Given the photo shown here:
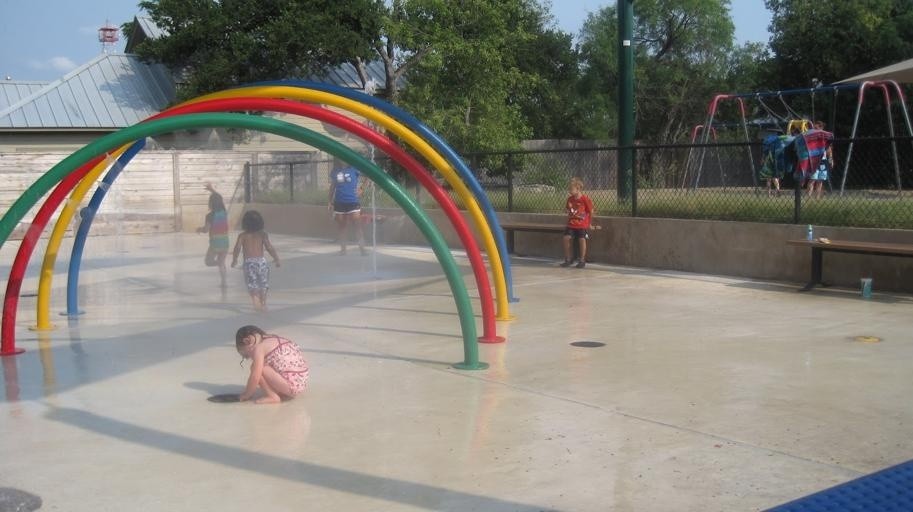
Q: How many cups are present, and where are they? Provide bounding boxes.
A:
[860,278,873,298]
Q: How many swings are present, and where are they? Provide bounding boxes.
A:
[721,125,741,185]
[756,90,837,170]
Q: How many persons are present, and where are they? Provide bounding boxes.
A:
[236,326,310,405]
[758,116,833,201]
[327,155,368,255]
[198,182,230,301]
[231,209,280,311]
[561,178,593,269]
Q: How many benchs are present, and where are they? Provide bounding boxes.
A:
[497,222,603,262]
[786,238,913,292]
[328,214,386,242]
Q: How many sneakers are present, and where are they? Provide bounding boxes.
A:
[560,262,571,267]
[576,262,585,269]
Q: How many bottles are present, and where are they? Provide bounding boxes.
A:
[807,224,813,240]
